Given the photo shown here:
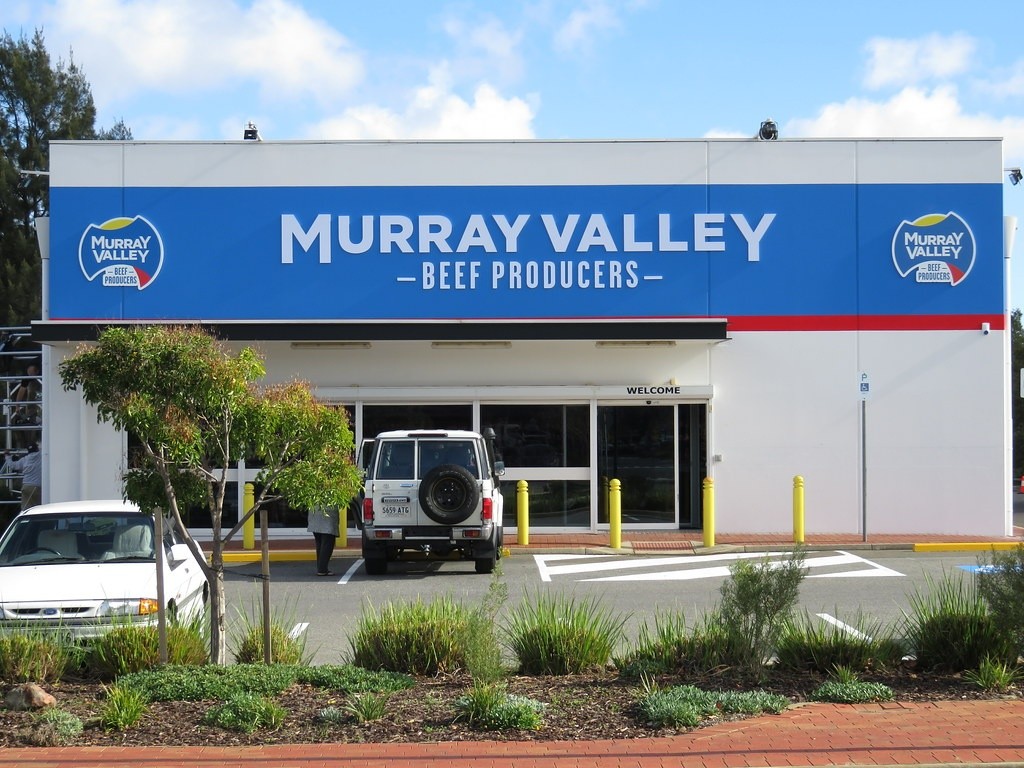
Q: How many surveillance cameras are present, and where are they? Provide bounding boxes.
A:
[762,125,775,139]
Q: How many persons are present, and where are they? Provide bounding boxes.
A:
[307,505,340,575]
[5,442,41,510]
[16,354,42,425]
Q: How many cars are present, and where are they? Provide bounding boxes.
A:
[0,500,210,652]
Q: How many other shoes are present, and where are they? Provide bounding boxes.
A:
[317,571,335,576]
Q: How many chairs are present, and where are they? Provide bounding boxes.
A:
[36,529,85,562]
[99,524,156,564]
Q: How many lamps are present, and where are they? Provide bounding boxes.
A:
[243,120,261,141]
[1004,167,1023,186]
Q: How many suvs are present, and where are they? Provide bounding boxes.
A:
[348,427,505,576]
